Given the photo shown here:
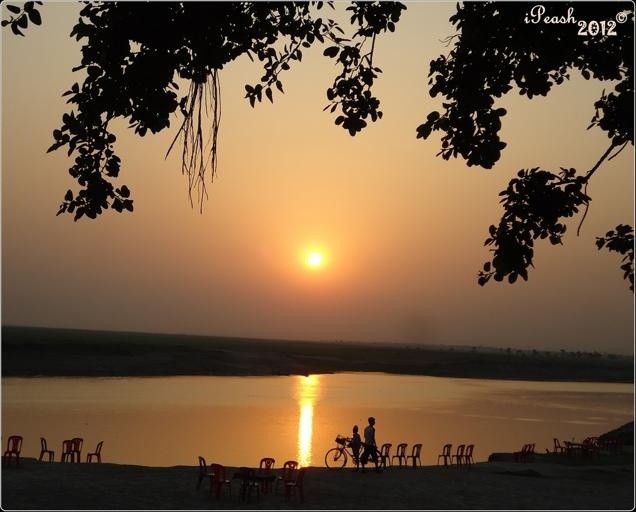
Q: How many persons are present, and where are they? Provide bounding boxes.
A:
[347,425,360,472]
[358,416,382,473]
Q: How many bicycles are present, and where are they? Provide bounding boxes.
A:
[325,435,383,469]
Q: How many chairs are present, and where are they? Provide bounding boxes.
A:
[238,467,260,504]
[208,463,232,500]
[407,444,423,468]
[380,444,392,468]
[286,468,305,504]
[260,458,275,495]
[462,445,474,465]
[438,444,452,466]
[1,434,104,464]
[392,443,408,468]
[275,461,298,496]
[196,456,214,490]
[510,437,622,464]
[452,445,465,464]
